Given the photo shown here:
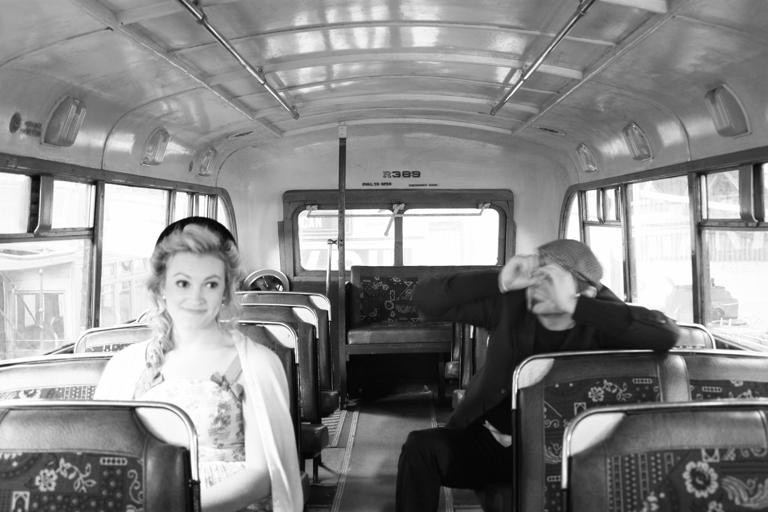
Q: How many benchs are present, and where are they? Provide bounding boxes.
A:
[444,294,765,512]
[0,265,330,512]
[340,266,511,396]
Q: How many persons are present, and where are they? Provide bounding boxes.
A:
[391,237,682,512]
[90,219,305,511]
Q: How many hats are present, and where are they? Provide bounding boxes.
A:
[153,215,236,251]
[537,239,603,291]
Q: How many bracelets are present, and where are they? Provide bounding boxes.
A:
[499,269,509,293]
